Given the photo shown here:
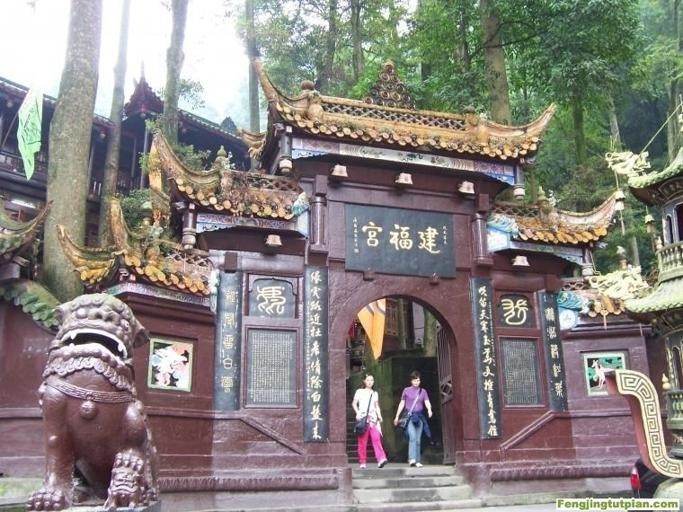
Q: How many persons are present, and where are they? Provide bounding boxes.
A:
[392,370,432,467]
[350,372,388,469]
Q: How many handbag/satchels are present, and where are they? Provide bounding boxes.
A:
[397,412,410,430]
[355,417,366,433]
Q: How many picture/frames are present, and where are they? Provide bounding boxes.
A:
[584,354,625,396]
[147,338,193,393]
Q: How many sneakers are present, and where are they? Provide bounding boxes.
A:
[360,464,366,469]
[377,459,387,468]
[409,459,423,468]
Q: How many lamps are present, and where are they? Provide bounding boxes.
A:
[263,232,282,248]
[329,162,476,196]
[510,254,530,267]
